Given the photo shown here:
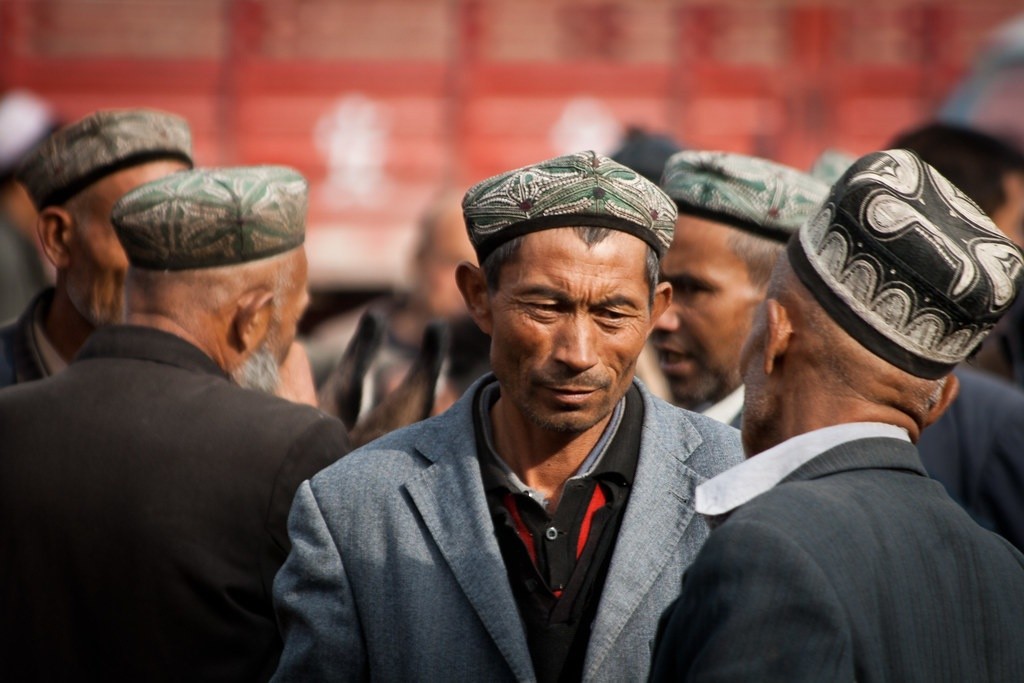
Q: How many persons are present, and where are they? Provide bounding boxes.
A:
[0,89,1023,543]
[646,147,1024,683]
[0,161,353,683]
[269,150,746,683]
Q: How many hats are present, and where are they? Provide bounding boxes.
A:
[14,111,193,212]
[655,149,831,243]
[0,90,63,180]
[787,147,1024,380]
[463,150,678,266]
[111,165,308,270]
